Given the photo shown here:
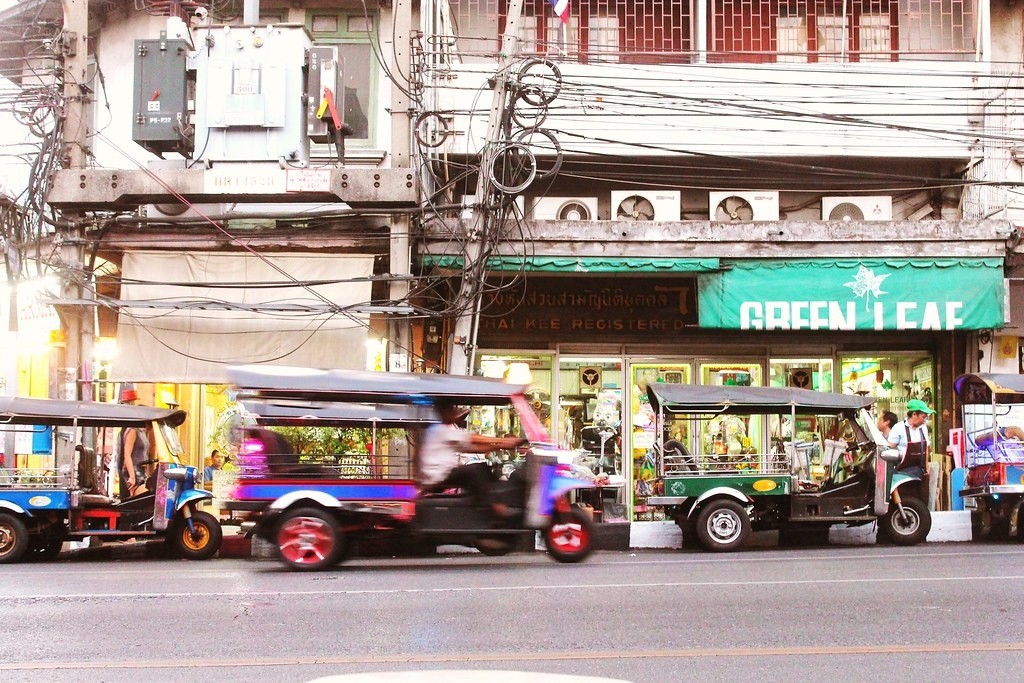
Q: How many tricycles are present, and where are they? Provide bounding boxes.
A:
[951,372,1023,545]
[0,395,224,563]
[226,360,605,571]
[645,382,933,553]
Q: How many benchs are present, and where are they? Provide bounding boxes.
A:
[664,439,698,476]
[251,426,338,479]
[976,426,1024,464]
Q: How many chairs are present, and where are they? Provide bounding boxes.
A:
[776,440,819,487]
[75,445,117,504]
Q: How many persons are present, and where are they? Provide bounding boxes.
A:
[875,399,937,546]
[418,408,525,518]
[877,410,898,442]
[118,406,152,544]
[204,450,240,482]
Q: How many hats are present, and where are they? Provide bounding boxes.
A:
[907,399,938,415]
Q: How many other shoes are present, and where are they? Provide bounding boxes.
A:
[499,507,523,516]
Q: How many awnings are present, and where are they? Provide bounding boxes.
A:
[113,251,370,383]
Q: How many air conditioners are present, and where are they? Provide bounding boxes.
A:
[531,196,598,223]
[460,194,524,221]
[820,195,892,222]
[609,190,681,224]
[708,190,779,224]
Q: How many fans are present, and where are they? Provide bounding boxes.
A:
[525,388,551,421]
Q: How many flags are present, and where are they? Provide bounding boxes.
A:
[549,0,570,24]
